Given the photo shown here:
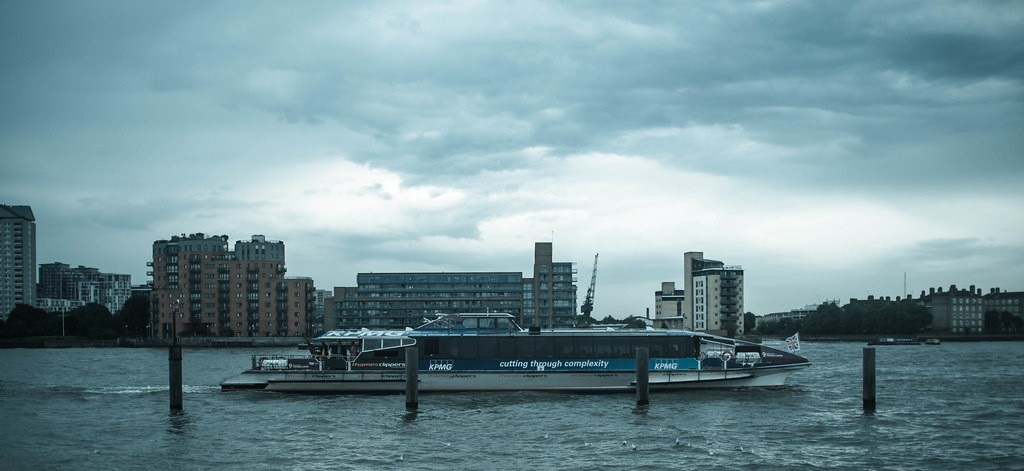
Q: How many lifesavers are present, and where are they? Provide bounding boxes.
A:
[258,356,268,367]
[722,351,733,362]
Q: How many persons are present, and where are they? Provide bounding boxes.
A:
[307,341,362,362]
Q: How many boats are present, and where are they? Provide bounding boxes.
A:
[926,338,940,345]
[220,307,811,393]
[867,336,922,345]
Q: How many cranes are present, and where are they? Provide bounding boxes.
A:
[581,253,600,320]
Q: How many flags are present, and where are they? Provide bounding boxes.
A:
[784,332,800,352]
